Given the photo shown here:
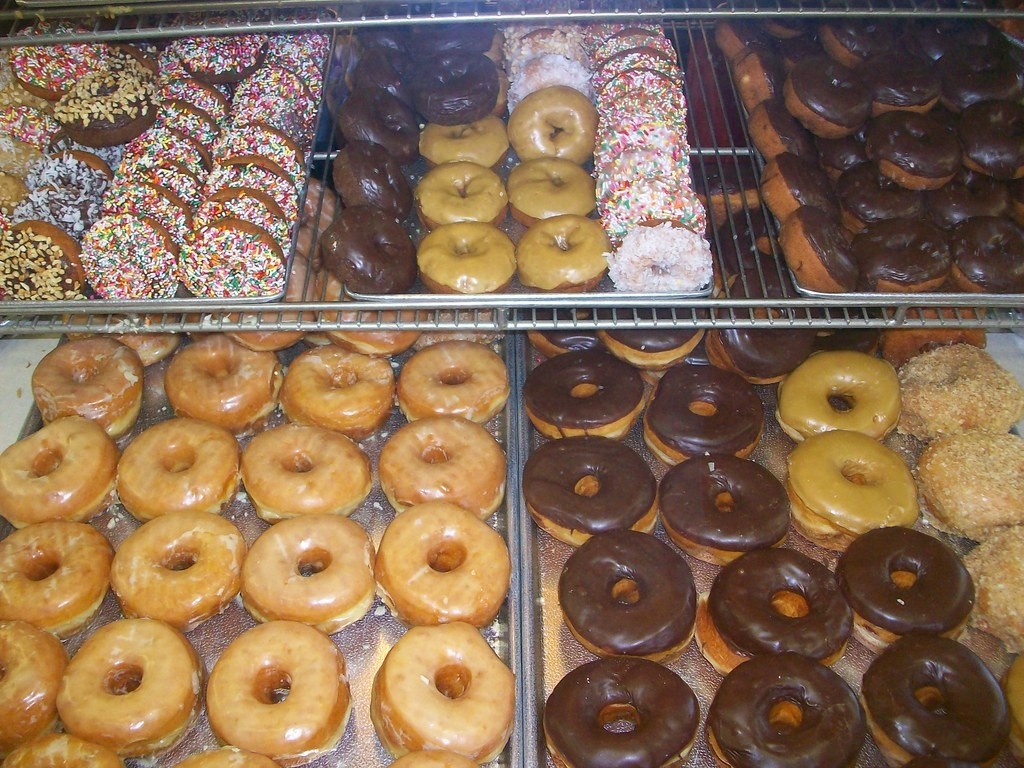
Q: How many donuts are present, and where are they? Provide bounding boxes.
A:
[0,0,1024,768]
